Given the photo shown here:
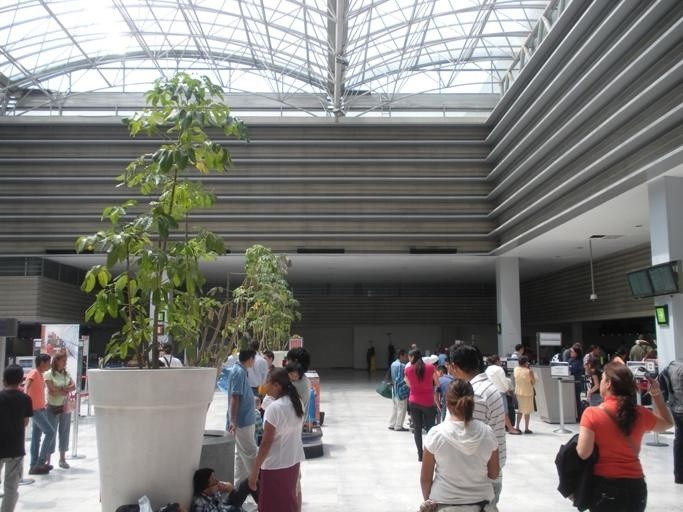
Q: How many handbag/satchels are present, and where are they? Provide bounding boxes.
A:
[510,394,517,409]
[375,381,394,398]
[62,389,76,413]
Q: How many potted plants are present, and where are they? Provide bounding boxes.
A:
[233,244,303,370]
[72,72,252,512]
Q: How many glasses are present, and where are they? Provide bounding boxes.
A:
[631,367,657,379]
[203,479,219,489]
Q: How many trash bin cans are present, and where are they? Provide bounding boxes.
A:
[197,429,236,486]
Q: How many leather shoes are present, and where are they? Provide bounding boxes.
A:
[395,426,409,431]
[389,426,394,430]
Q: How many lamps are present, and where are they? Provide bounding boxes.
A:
[589,239,598,303]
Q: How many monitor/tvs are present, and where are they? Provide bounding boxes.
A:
[656,306,668,323]
[648,260,679,296]
[626,269,654,298]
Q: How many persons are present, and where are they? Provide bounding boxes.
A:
[513,356,535,434]
[44,352,75,469]
[419,377,500,511]
[667,357,683,483]
[24,353,56,475]
[510,343,524,359]
[484,354,522,434]
[0,363,33,512]
[159,341,183,367]
[562,332,657,408]
[575,360,672,512]
[189,467,260,512]
[227,339,312,512]
[387,343,455,462]
[450,343,508,510]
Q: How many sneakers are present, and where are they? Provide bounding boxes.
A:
[417,452,423,461]
[18,477,35,485]
[59,460,68,468]
[29,466,49,473]
[675,477,683,483]
[406,419,427,433]
[0,483,5,497]
[33,463,52,471]
[509,428,532,435]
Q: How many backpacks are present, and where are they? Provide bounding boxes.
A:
[657,360,678,406]
[395,362,409,401]
